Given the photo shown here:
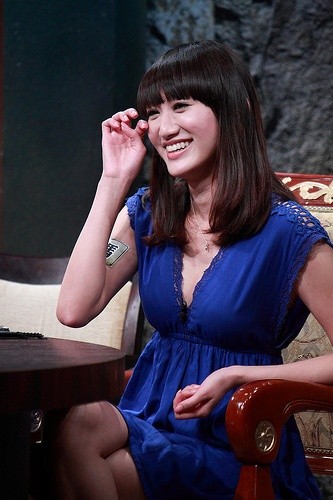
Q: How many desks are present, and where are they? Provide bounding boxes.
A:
[0,337,126,500]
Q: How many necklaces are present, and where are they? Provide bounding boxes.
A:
[189,196,212,251]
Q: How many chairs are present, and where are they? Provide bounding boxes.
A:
[121,173,333,500]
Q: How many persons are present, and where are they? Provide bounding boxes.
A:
[48,30,333,500]
